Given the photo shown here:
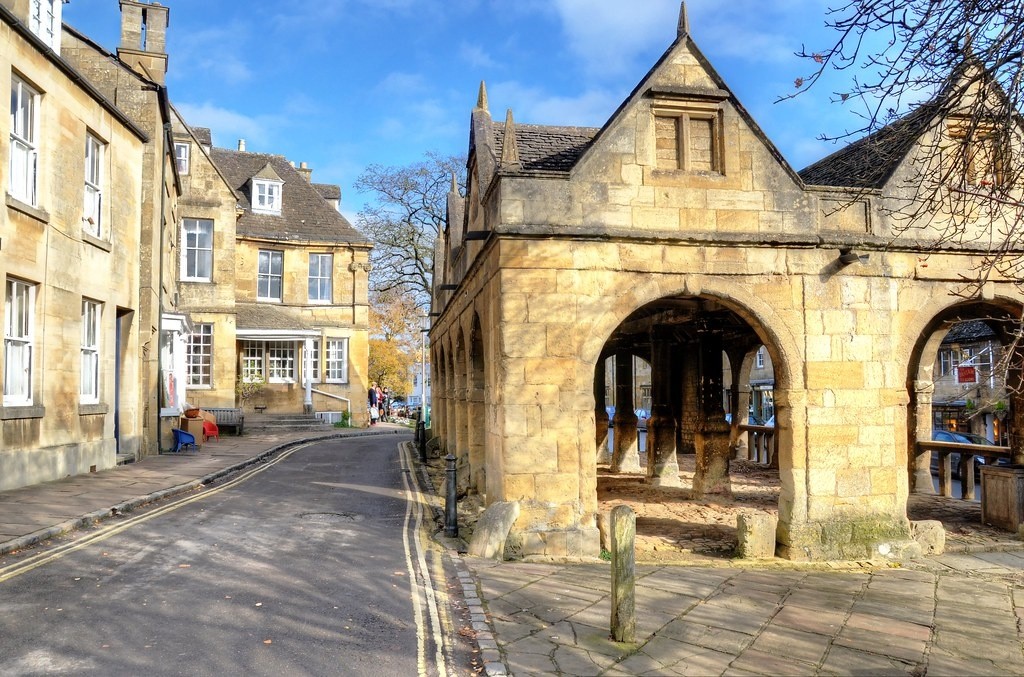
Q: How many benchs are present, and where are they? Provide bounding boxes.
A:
[197,407,244,435]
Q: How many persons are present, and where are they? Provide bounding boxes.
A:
[368,382,394,426]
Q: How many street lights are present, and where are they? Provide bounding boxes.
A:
[418,312,429,424]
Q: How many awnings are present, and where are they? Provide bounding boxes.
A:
[751,378,774,388]
[932,397,967,408]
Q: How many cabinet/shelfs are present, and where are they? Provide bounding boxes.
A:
[181,417,203,446]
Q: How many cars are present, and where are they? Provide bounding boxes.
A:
[634,409,652,432]
[604,406,617,427]
[391,401,431,420]
[931,429,1008,483]
[725,413,761,447]
[761,415,777,448]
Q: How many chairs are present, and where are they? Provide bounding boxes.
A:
[171,428,195,453]
[203,419,219,443]
